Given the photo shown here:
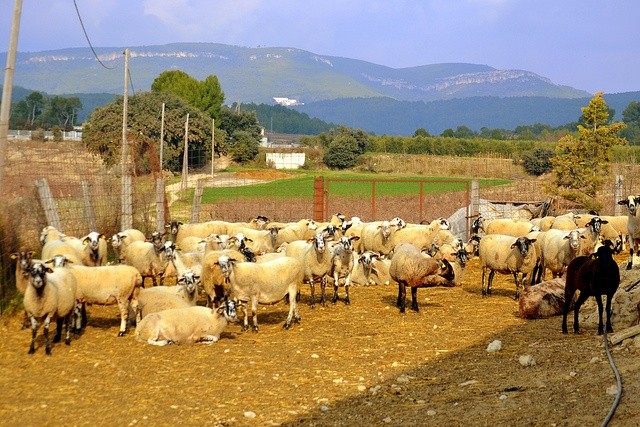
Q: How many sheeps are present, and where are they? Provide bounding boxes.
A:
[559,245,619,337]
[576,215,622,252]
[517,262,585,320]
[600,215,629,252]
[473,214,539,238]
[531,211,560,236]
[575,208,605,328]
[554,212,582,235]
[476,235,540,301]
[534,229,587,284]
[10,213,479,357]
[617,195,640,270]
